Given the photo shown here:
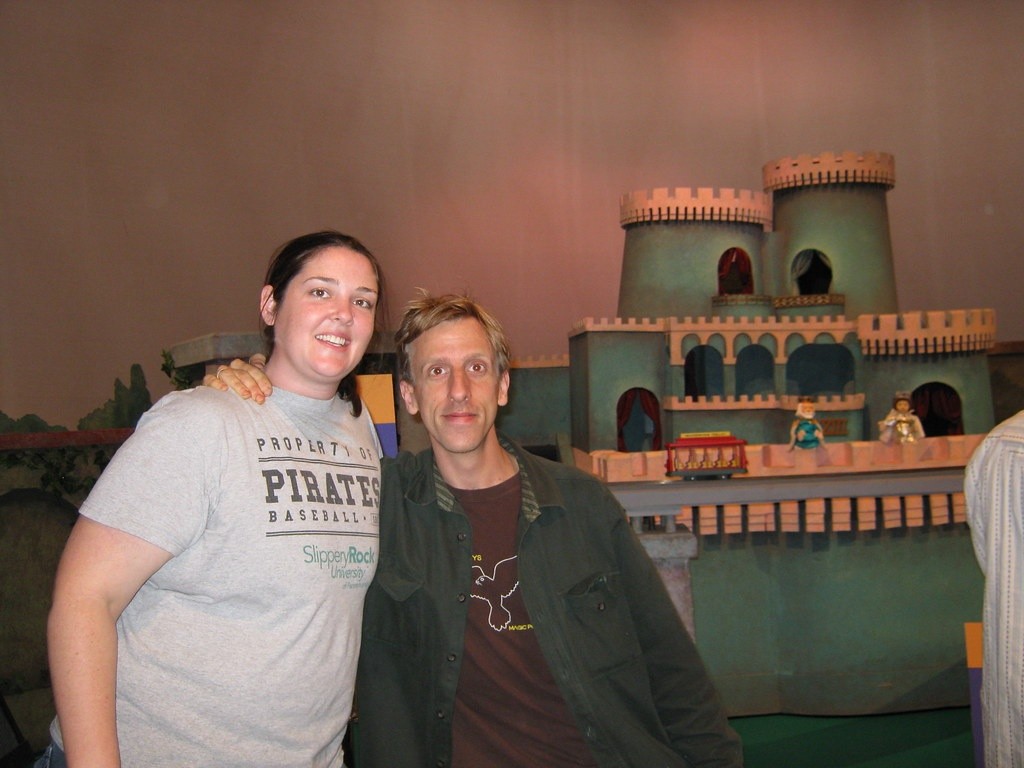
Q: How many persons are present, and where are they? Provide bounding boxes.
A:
[964,408,1024,768]
[786,395,827,452]
[876,391,926,446]
[202,288,740,768]
[46,229,384,768]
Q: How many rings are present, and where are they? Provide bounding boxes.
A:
[215,367,229,379]
